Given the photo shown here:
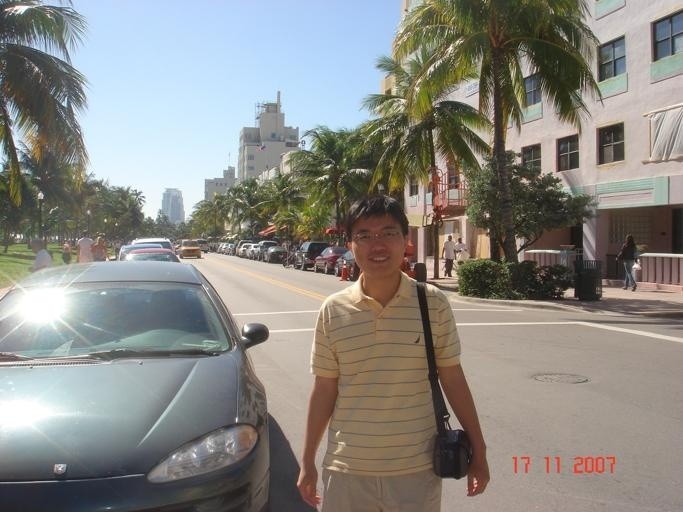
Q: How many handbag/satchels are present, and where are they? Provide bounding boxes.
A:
[433,428,472,479]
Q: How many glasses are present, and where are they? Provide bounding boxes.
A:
[353,229,404,243]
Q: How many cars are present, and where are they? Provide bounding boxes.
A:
[0,259,270,512]
[115,234,411,281]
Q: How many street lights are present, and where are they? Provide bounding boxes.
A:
[36,190,122,265]
[430,196,443,280]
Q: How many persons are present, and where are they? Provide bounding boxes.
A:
[28,233,137,273]
[616,234,639,292]
[441,235,456,277]
[295,196,491,512]
[454,237,468,266]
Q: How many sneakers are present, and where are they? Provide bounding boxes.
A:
[621,283,637,292]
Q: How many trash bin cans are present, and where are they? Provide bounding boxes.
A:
[572,260,603,302]
